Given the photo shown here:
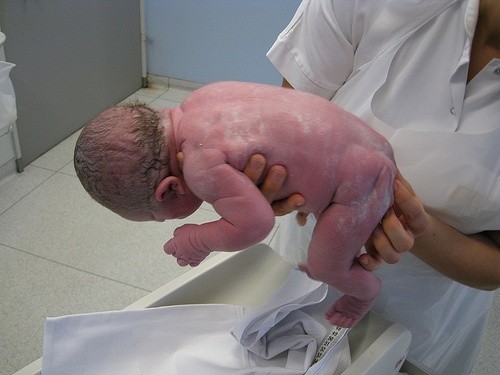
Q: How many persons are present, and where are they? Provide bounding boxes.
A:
[230,0,500,375]
[72,79,399,331]
